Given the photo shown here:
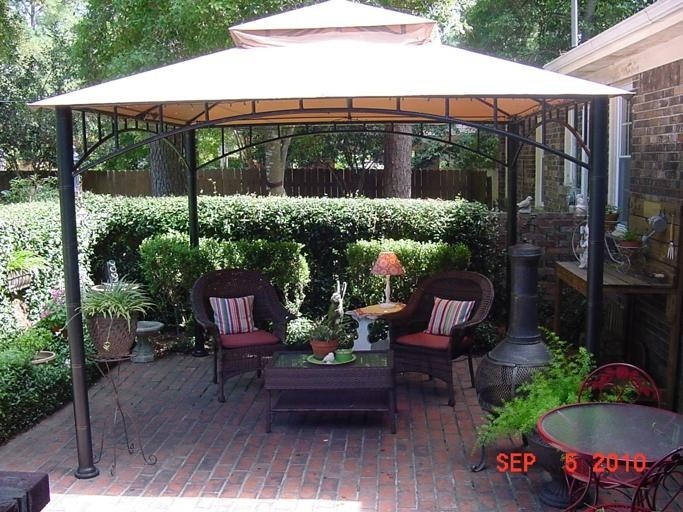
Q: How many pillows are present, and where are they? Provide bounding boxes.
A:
[208,295,258,334]
[424,295,475,337]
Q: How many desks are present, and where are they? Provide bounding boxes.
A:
[537,401,683,512]
[554,261,683,411]
[345,303,407,351]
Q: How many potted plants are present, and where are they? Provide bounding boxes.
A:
[470,326,650,509]
[604,204,641,256]
[282,317,354,362]
[4,250,44,293]
[58,272,157,360]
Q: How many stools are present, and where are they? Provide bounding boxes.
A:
[130,321,165,363]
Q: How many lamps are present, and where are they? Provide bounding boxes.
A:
[372,252,404,308]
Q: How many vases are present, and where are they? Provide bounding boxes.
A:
[31,350,55,364]
[52,326,67,339]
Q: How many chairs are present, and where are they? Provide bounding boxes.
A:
[192,268,292,402]
[564,363,661,512]
[587,446,683,512]
[388,271,495,406]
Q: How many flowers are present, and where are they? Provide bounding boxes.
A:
[40,287,67,328]
